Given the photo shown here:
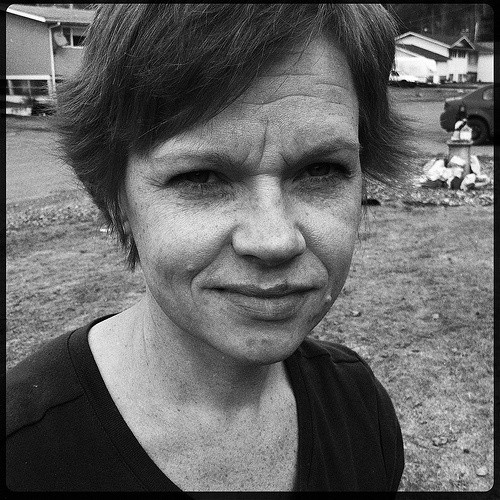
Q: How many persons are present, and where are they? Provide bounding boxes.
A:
[6,5,420,492]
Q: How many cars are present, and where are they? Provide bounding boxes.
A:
[439,85,494,145]
